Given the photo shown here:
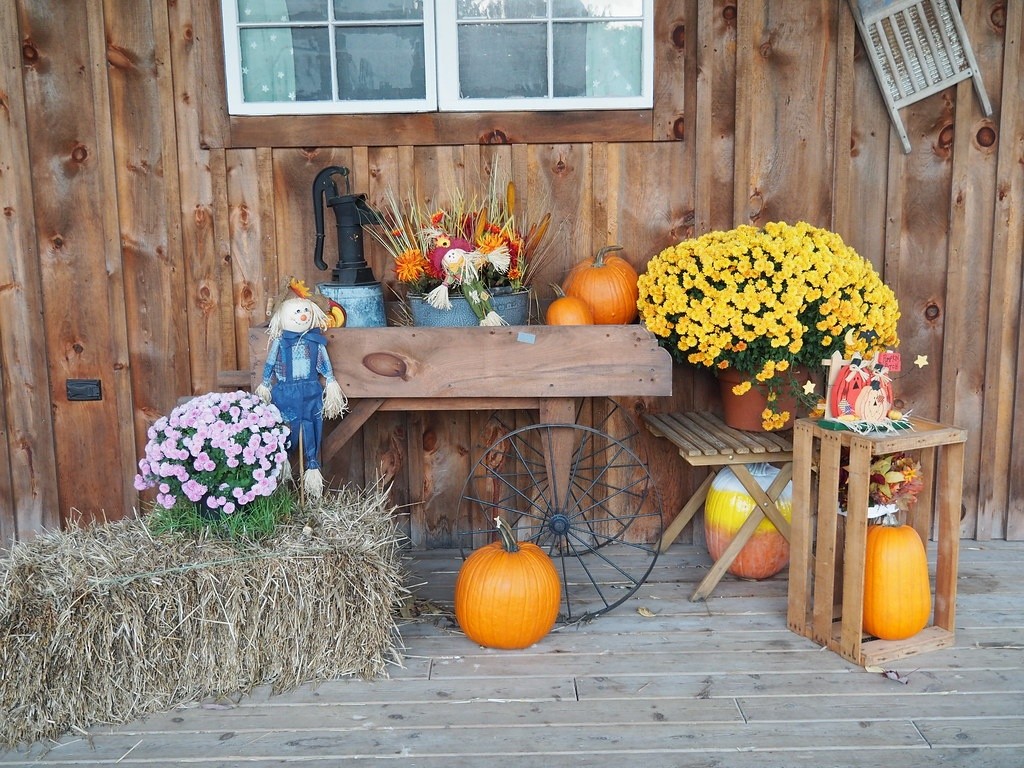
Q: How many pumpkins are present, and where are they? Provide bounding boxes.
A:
[545,245,642,326]
[863,518,933,639]
[705,462,792,578]
[454,516,563,650]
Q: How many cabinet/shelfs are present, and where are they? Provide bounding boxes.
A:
[642,406,793,603]
[787,411,970,669]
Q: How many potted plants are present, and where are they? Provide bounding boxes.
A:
[134,390,292,519]
[354,151,572,327]
[837,446,924,527]
[635,217,904,432]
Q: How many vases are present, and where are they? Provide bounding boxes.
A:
[319,280,387,326]
